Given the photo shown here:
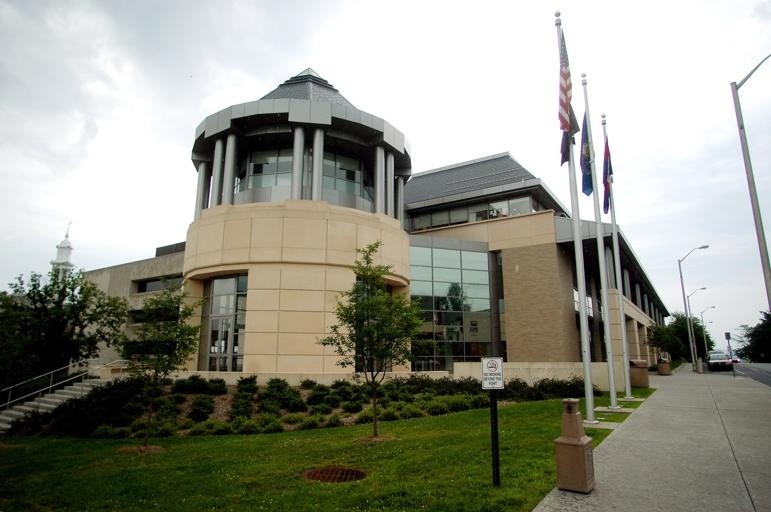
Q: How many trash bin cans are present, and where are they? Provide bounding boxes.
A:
[657,358,670,375]
[696,357,703,373]
[629,359,649,388]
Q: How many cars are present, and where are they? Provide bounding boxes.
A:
[707,349,741,373]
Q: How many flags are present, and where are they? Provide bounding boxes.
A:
[602,137,614,213]
[556,27,579,165]
[580,113,593,196]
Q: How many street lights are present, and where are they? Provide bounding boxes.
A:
[677,244,717,371]
